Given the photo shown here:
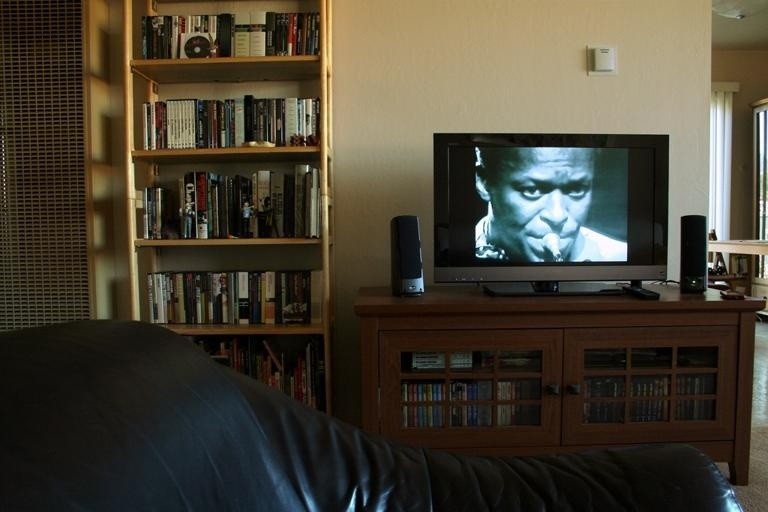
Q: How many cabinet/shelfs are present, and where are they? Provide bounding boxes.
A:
[120,0,333,419]
[354,285,767,489]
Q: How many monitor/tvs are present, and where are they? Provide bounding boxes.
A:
[434,132,669,296]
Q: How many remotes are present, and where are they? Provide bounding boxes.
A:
[624,286,659,300]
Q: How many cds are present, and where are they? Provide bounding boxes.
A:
[184,37,211,58]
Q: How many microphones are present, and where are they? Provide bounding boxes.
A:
[542,233,563,263]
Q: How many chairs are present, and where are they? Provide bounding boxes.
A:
[708,229,748,291]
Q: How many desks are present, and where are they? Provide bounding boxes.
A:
[708,239,768,256]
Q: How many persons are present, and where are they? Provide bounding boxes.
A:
[474,147,628,263]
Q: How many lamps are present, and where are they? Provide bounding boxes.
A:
[712,0,768,19]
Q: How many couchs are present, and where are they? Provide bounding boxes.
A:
[1,318,747,512]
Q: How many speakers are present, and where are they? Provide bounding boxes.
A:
[680,215,707,294]
[391,215,424,297]
